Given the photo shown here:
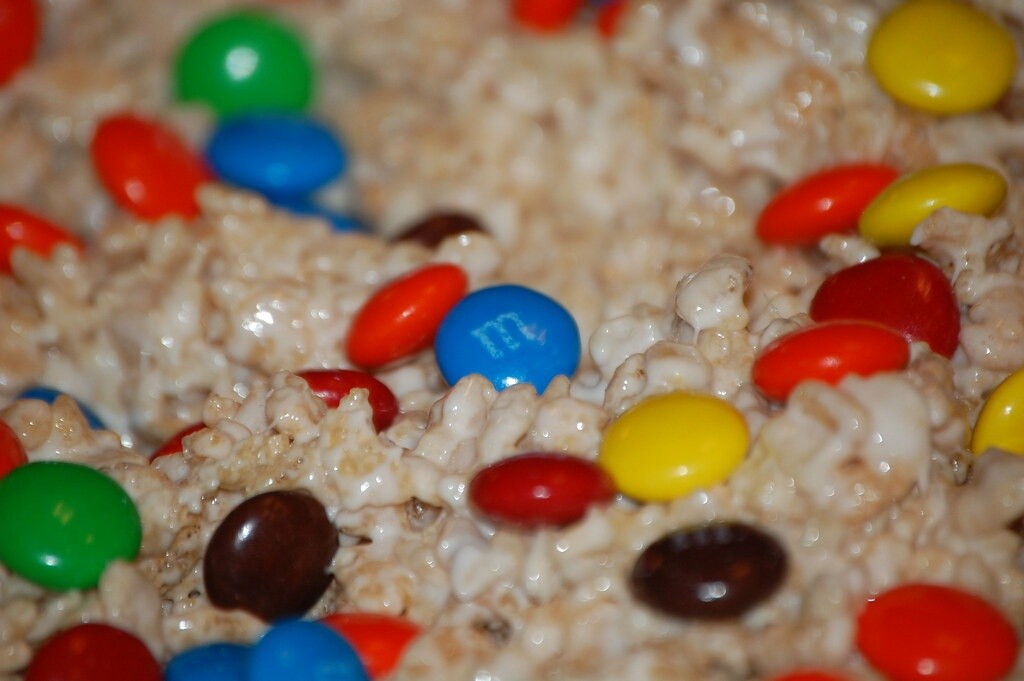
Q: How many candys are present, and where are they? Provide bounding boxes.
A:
[1,0,1024,681]
